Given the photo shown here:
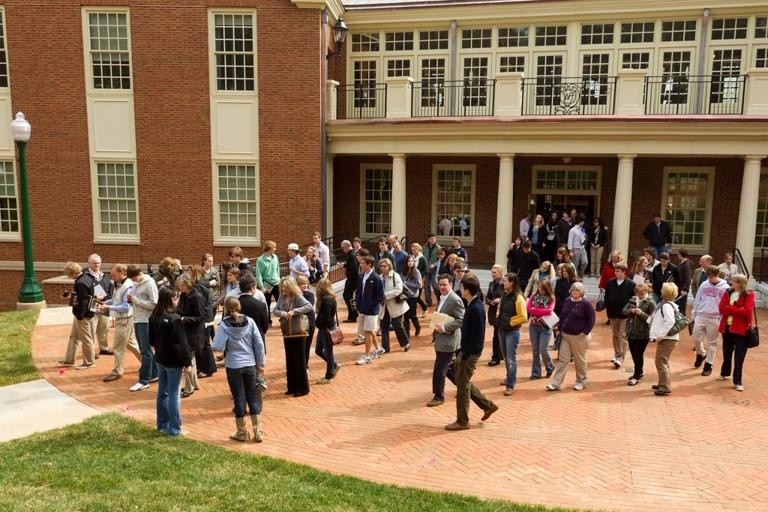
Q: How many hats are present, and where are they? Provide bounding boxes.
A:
[288,243,300,251]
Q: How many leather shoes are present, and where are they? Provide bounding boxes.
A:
[285,389,309,397]
[103,373,122,383]
[628,374,643,386]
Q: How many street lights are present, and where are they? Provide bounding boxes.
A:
[7,108,48,312]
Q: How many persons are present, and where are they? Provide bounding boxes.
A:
[643,213,672,259]
[59,254,141,381]
[520,208,607,281]
[126,254,220,436]
[691,253,755,390]
[485,237,596,395]
[342,235,429,365]
[423,233,499,430]
[256,233,341,396]
[213,247,268,441]
[440,214,470,236]
[599,248,693,395]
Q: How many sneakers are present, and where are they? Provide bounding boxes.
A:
[445,421,471,430]
[716,372,729,380]
[57,347,114,370]
[156,426,189,437]
[694,353,706,367]
[480,404,499,421]
[609,355,624,367]
[573,380,587,390]
[488,355,555,396]
[590,272,600,277]
[426,396,444,407]
[129,376,159,391]
[652,385,671,396]
[181,353,225,398]
[342,309,428,366]
[736,384,743,391]
[316,364,341,384]
[547,382,560,390]
[702,362,712,376]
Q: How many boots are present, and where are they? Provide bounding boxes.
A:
[250,413,266,443]
[230,416,249,442]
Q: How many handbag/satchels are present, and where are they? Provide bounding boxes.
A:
[595,300,606,312]
[328,327,343,345]
[537,310,560,331]
[310,271,323,283]
[394,281,419,303]
[280,313,309,337]
[745,326,759,348]
[665,313,690,336]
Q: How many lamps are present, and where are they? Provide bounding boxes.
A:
[326,15,349,60]
